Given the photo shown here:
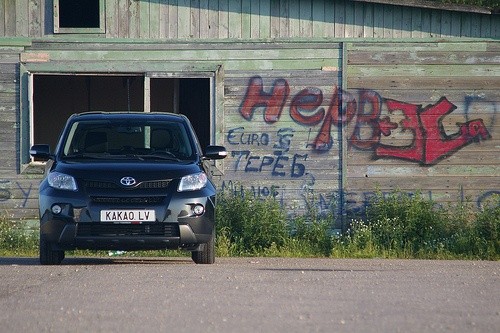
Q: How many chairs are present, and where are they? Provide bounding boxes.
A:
[82,132,108,154]
[150,128,172,152]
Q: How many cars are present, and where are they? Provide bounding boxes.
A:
[28,110,228,264]
